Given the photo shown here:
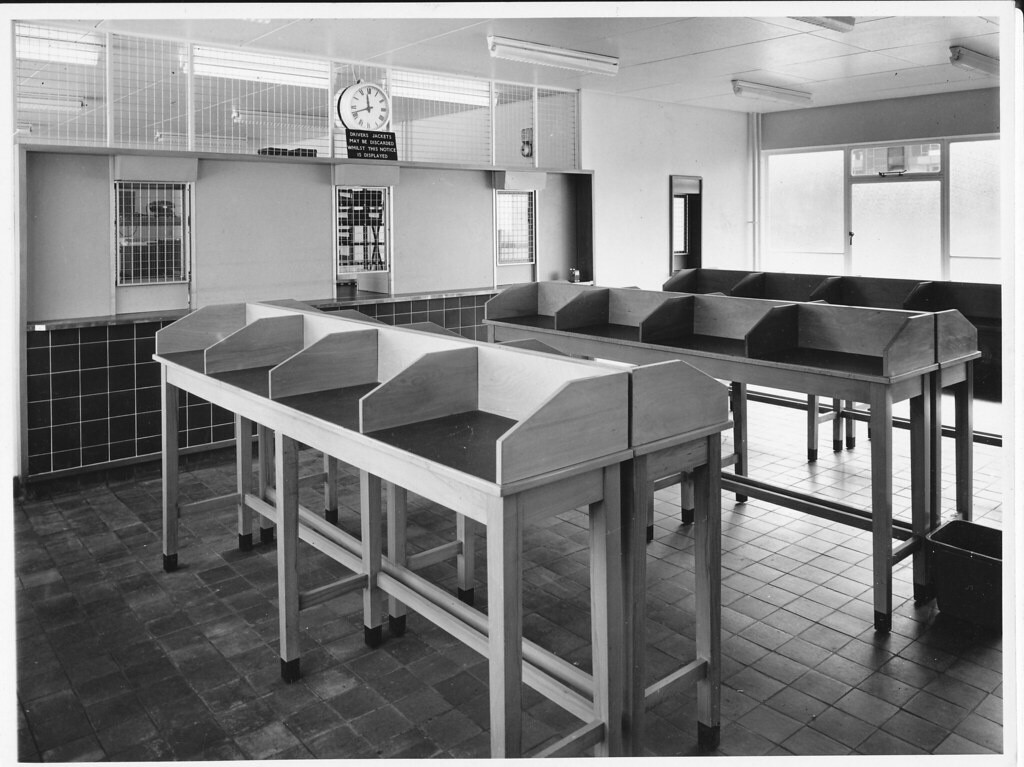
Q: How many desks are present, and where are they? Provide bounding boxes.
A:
[152,265,1002,756]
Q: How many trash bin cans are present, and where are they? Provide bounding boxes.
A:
[925,518,1002,634]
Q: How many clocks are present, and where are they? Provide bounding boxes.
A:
[338,78,390,132]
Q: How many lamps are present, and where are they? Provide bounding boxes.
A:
[155,130,249,149]
[15,122,34,134]
[485,36,618,79]
[229,111,329,133]
[948,44,999,80]
[16,93,86,116]
[731,79,811,108]
[791,17,855,37]
